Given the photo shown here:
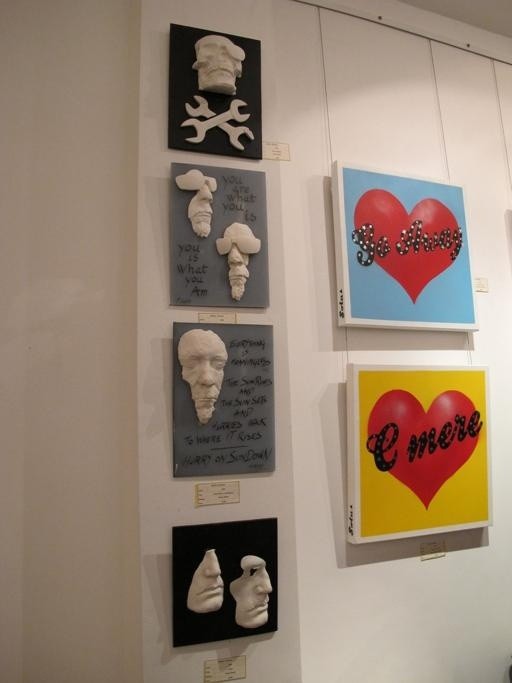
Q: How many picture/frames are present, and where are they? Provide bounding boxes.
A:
[332,160,480,332]
[346,364,495,544]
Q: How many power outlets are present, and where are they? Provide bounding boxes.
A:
[476,277,488,292]
